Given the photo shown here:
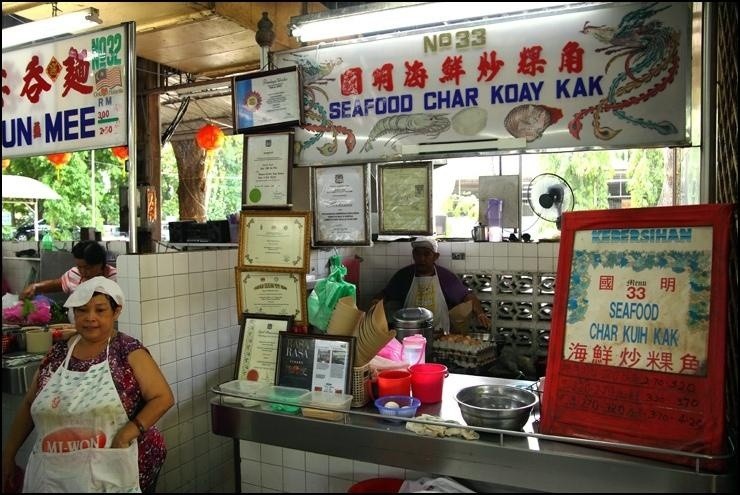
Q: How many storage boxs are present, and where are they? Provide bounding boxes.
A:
[258,385,309,416]
[295,390,354,422]
[220,378,267,409]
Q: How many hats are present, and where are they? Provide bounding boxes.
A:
[411,234,439,255]
[63,276,125,315]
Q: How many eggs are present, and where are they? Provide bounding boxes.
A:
[438,333,482,356]
[400,405,412,417]
[382,400,399,416]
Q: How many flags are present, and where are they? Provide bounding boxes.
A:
[93,67,122,90]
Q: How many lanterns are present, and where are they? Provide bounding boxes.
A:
[47,153,73,181]
[113,146,130,177]
[197,124,225,176]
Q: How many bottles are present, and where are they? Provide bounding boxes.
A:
[400,332,427,367]
[41,231,53,253]
[43,325,49,333]
[471,221,489,242]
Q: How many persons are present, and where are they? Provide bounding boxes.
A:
[369,236,490,335]
[1,276,175,493]
[20,239,117,299]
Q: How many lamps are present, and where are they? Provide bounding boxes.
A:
[2,0,103,53]
[285,1,593,46]
[48,152,71,167]
[112,145,128,160]
[195,122,225,151]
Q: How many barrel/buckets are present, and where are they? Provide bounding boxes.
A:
[378,371,411,397]
[408,362,449,404]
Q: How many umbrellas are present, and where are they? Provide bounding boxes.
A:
[2,175,61,203]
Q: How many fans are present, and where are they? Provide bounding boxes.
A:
[526,172,575,231]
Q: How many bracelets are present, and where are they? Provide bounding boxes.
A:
[475,313,485,320]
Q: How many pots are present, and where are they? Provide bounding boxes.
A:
[6,325,45,350]
[392,307,434,358]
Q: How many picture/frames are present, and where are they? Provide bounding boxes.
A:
[309,163,372,248]
[274,330,357,395]
[231,67,304,134]
[233,312,295,384]
[376,161,434,238]
[241,125,294,210]
[237,210,311,274]
[234,265,309,327]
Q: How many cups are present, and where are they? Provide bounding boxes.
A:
[488,198,503,243]
[368,371,412,407]
[229,223,240,242]
[26,329,51,353]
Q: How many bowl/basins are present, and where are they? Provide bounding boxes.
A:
[48,322,78,342]
[455,385,540,435]
